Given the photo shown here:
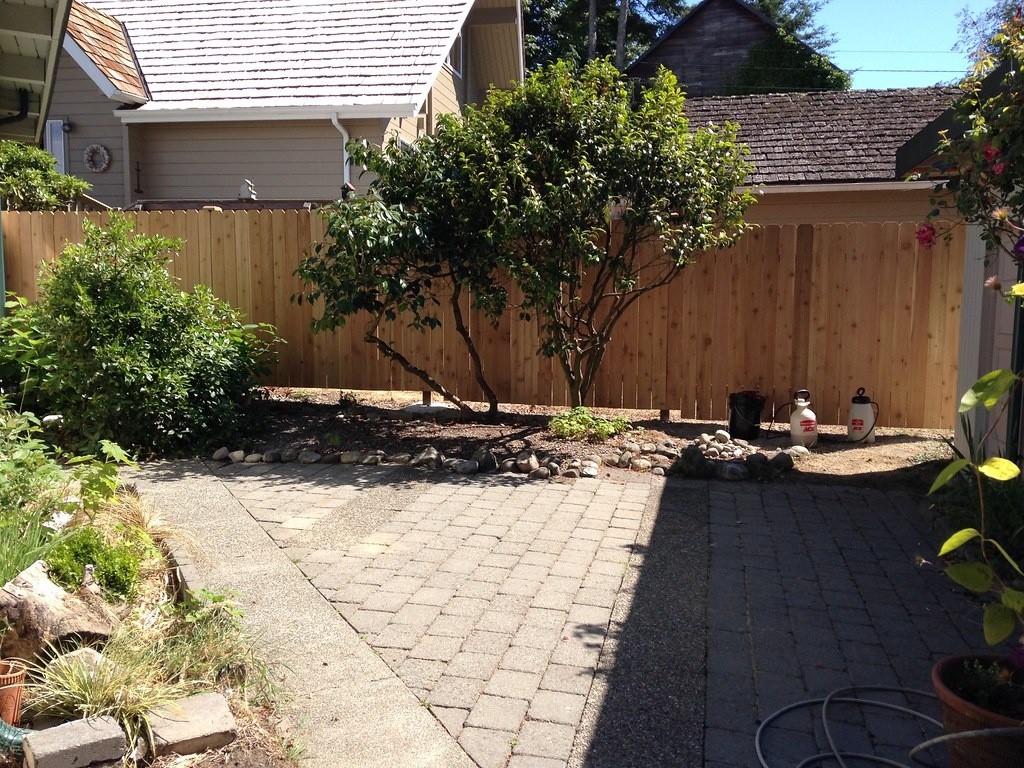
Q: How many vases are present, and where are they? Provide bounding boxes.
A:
[0,660,27,726]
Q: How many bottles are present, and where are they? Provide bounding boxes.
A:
[847,387,875,443]
[790,389,818,448]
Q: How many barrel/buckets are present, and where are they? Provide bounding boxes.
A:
[0,661,27,727]
[730,389,765,440]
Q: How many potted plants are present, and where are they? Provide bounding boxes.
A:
[924,367,1024,768]
